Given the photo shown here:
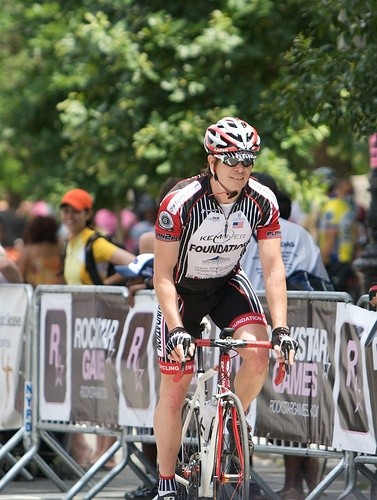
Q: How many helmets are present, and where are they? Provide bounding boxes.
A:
[204,117,260,155]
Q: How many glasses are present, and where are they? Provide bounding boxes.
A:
[214,155,256,167]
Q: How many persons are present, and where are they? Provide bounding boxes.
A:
[154,117,299,500]
[0,166,377,483]
[369,133,377,240]
[58,188,137,470]
[237,172,334,500]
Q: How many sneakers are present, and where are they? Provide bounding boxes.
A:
[151,490,177,500]
[223,411,254,456]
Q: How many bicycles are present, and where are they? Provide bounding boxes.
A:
[168,327,286,499]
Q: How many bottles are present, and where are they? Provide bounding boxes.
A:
[199,395,218,444]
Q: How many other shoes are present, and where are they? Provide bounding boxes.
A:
[262,486,306,500]
[303,457,327,490]
[0,448,115,472]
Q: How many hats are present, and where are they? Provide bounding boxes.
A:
[116,253,154,275]
[369,286,377,294]
[59,189,92,210]
